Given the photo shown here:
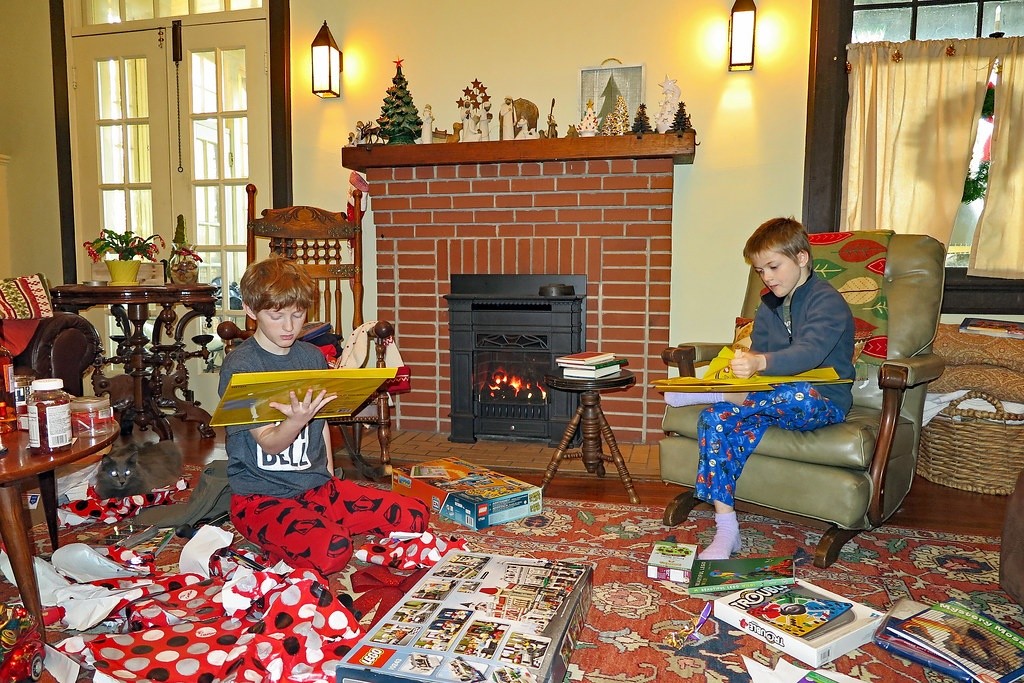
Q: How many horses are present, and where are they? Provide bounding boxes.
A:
[567,124,578,138]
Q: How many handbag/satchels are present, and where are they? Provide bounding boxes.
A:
[134,460,230,527]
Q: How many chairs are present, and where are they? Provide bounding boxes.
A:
[217,183,393,467]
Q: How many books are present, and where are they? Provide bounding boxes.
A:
[887,599,1024,683]
[959,318,1024,339]
[83,522,175,557]
[557,352,629,378]
[689,555,796,595]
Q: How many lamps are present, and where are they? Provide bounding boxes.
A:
[311,20,343,99]
[728,0,756,71]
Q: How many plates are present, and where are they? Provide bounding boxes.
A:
[83,280,108,286]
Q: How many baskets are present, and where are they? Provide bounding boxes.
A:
[915,390,1024,494]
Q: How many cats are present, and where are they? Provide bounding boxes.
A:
[95,441,181,498]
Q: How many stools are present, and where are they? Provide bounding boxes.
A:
[542,367,640,504]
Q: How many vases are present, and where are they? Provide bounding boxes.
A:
[104,260,140,287]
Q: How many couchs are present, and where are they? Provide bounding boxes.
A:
[660,227,946,567]
[0,274,100,398]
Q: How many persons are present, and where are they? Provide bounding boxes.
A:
[697,217,855,560]
[218,260,430,573]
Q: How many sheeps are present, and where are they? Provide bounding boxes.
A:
[361,121,386,144]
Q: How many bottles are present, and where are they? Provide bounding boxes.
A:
[0,319,16,433]
[71,396,113,436]
[170,242,198,285]
[27,378,73,454]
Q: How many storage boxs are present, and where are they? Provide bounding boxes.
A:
[391,457,542,531]
[90,262,166,286]
[646,539,698,583]
[715,579,887,669]
[336,548,595,683]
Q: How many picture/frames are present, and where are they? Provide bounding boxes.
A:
[579,63,645,136]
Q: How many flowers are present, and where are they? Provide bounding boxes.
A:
[83,229,165,262]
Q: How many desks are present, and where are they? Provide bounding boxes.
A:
[50,284,216,444]
[0,419,120,643]
